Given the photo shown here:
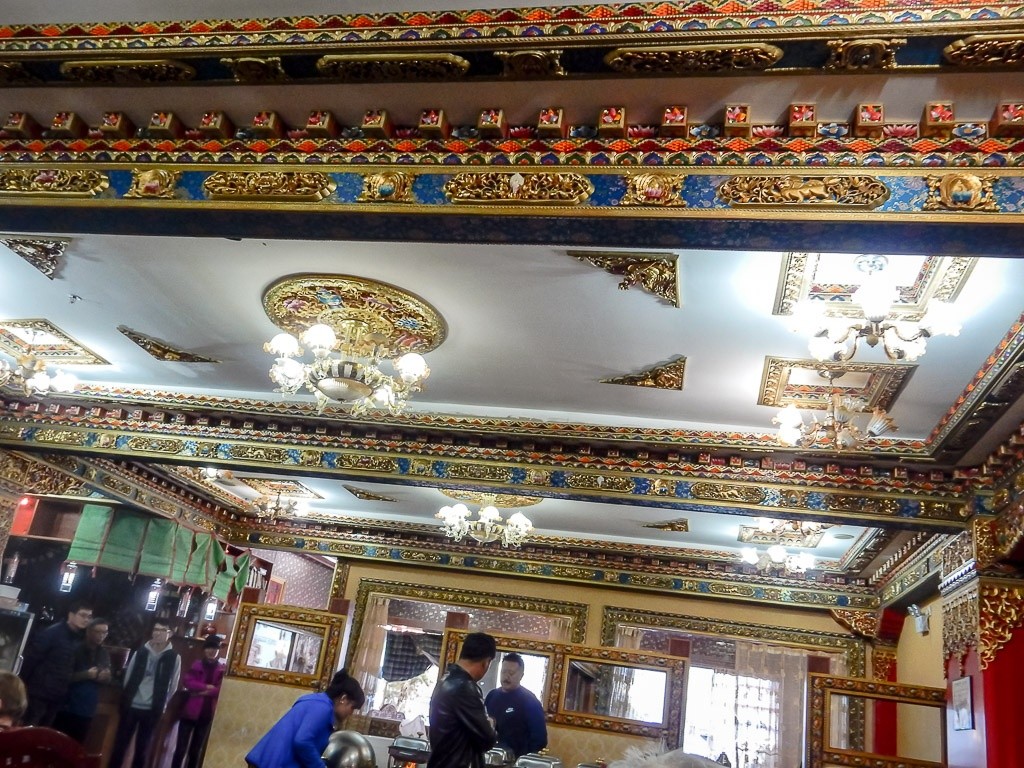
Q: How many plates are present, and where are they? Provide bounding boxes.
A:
[368,704,406,721]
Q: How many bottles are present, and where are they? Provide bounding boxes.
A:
[184,621,217,639]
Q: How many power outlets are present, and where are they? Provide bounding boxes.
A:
[926,606,931,616]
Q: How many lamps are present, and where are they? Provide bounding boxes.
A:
[176,587,192,618]
[786,274,966,368]
[771,367,898,449]
[60,561,78,593]
[435,492,533,548]
[756,513,824,540]
[197,467,225,484]
[254,480,308,522]
[203,595,218,621]
[0,328,80,397]
[145,577,162,612]
[741,544,817,575]
[263,320,431,417]
[907,604,929,633]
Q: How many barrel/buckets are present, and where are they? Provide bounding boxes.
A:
[100,645,131,676]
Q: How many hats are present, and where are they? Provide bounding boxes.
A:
[204,635,221,648]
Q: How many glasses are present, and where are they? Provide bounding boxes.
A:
[91,629,109,636]
[153,628,172,633]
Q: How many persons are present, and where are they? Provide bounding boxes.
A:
[244,669,365,768]
[51,619,112,740]
[427,632,516,768]
[170,635,225,768]
[483,651,548,763]
[18,602,93,740]
[0,673,28,733]
[106,617,181,768]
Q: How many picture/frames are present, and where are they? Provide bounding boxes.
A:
[951,675,974,731]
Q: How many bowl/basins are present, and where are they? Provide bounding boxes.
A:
[0,596,30,612]
[0,584,22,599]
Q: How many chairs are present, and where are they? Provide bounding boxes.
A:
[0,726,102,768]
